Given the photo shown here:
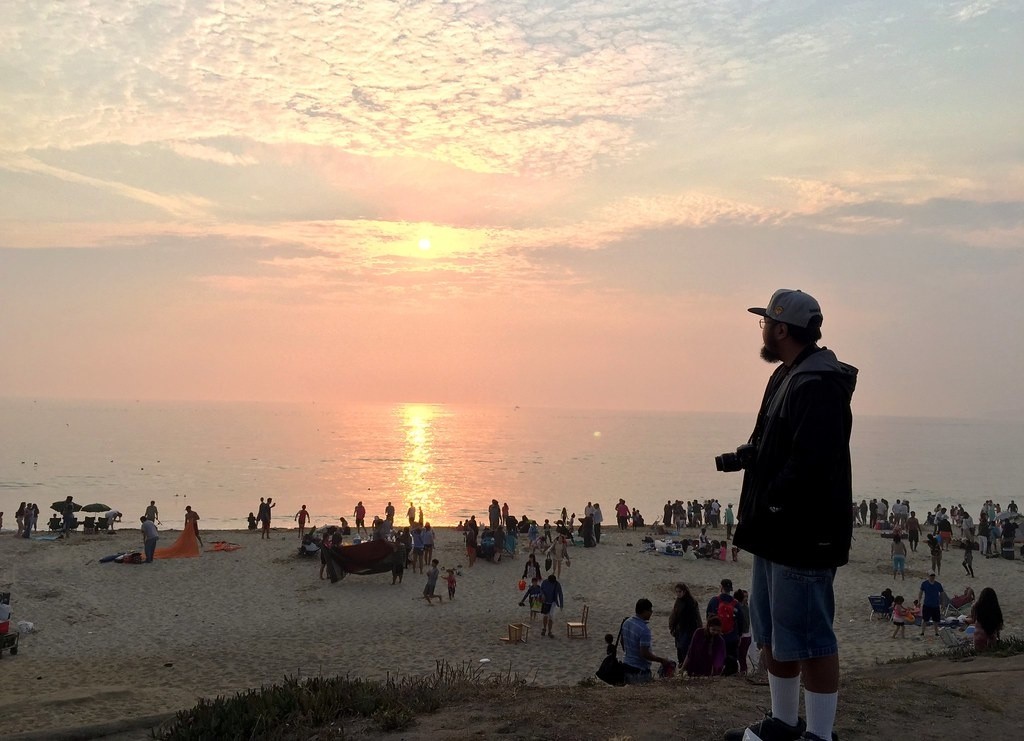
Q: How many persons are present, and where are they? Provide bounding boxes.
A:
[716,290,860,741]
[0,495,1024,687]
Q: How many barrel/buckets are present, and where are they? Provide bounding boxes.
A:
[519,580,526,590]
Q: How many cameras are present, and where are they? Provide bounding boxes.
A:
[714,443,758,473]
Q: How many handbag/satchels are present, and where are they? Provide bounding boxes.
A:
[596,654,630,686]
[519,579,526,590]
[545,558,552,570]
[531,595,543,612]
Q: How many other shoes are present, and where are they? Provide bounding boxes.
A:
[548,632,554,638]
[541,629,546,635]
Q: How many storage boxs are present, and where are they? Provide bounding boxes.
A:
[1003,547,1014,560]
[83,528,95,535]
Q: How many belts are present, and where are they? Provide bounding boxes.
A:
[625,665,651,675]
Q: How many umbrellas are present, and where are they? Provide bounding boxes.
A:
[50,501,82,514]
[82,504,111,518]
[994,511,1018,520]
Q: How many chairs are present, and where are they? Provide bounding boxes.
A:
[867,594,894,622]
[499,622,530,646]
[938,596,976,654]
[566,604,589,640]
[48,516,110,531]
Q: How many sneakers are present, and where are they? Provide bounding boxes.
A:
[798,731,839,741]
[723,704,806,741]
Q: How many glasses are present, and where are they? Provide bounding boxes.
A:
[759,319,779,328]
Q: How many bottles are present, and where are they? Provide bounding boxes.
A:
[361,537,368,542]
[565,530,584,547]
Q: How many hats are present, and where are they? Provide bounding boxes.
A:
[721,579,733,591]
[941,514,949,519]
[928,570,936,576]
[747,289,823,328]
